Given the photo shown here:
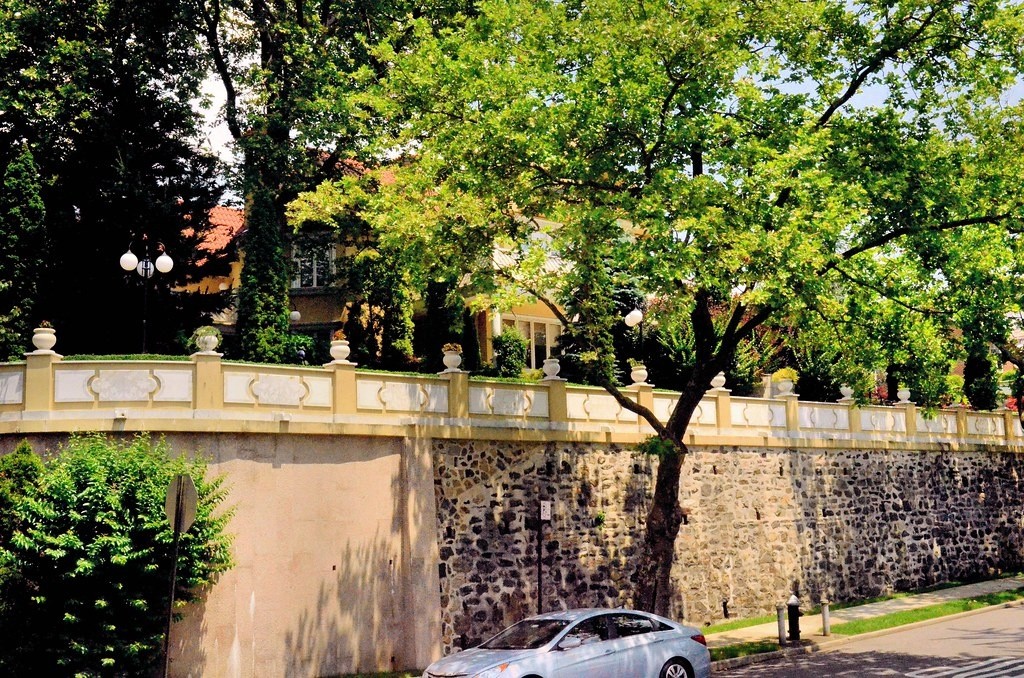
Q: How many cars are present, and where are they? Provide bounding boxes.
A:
[421,607,711,678]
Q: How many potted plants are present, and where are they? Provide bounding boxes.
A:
[771,363,800,394]
[186,322,224,352]
[839,377,857,398]
[541,355,561,379]
[896,381,912,402]
[948,385,964,407]
[992,391,1011,411]
[626,355,651,382]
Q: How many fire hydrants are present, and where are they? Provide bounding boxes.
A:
[786,595,805,641]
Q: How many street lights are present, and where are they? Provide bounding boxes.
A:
[626,305,644,362]
[119,234,175,354]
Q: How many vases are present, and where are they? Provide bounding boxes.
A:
[330,340,352,363]
[30,327,57,351]
[443,350,462,371]
[710,371,727,389]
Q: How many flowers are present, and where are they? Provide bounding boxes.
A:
[39,319,53,327]
[441,342,463,354]
[332,327,348,340]
